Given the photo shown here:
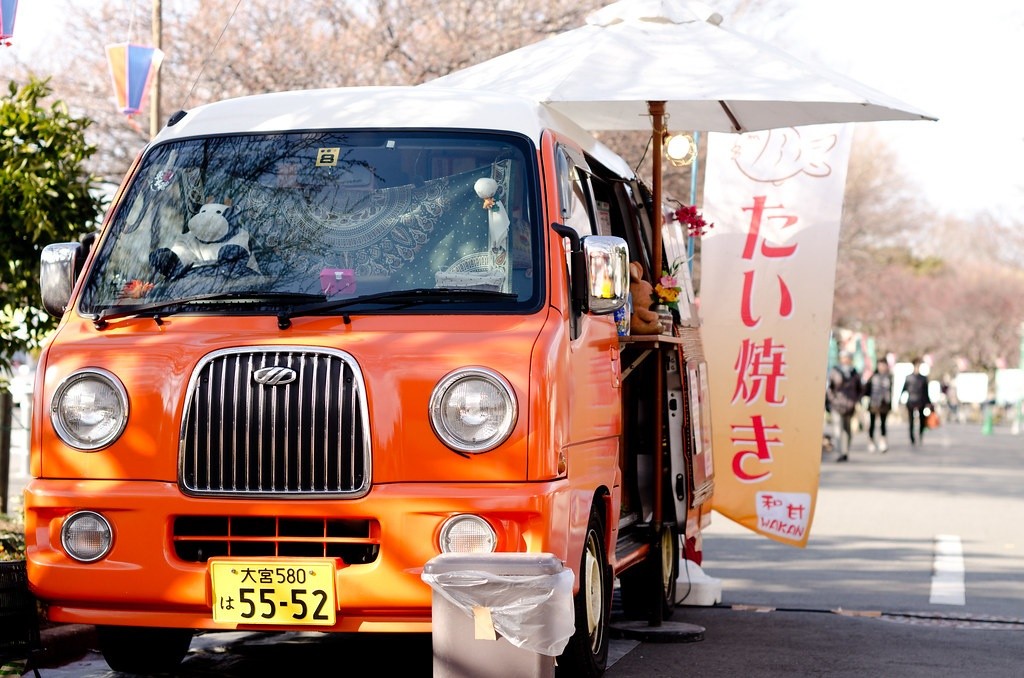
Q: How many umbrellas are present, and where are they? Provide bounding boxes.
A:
[412,0,937,536]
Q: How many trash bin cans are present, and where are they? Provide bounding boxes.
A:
[423,551,565,678]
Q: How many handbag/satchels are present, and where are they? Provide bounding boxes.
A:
[925,409,940,429]
[832,396,854,415]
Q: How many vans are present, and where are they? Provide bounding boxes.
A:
[23,81,715,678]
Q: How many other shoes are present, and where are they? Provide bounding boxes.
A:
[878,439,888,452]
[835,454,847,462]
[868,442,876,452]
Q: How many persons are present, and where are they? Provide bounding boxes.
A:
[822,350,936,462]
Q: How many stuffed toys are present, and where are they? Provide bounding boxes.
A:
[628,261,662,336]
[149,201,263,279]
